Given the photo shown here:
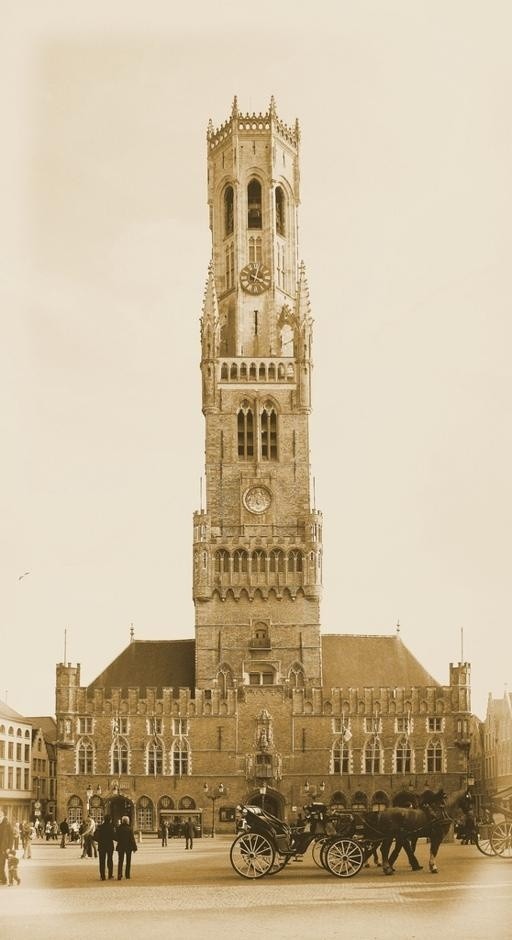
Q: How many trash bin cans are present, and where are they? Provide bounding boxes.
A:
[195,827,201,838]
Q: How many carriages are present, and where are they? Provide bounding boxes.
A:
[229,787,475,879]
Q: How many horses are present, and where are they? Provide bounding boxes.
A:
[361,786,477,876]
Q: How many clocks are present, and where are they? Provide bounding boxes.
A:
[240,262,271,295]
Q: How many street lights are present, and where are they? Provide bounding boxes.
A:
[409,776,430,792]
[203,783,224,838]
[260,783,267,810]
[304,780,325,802]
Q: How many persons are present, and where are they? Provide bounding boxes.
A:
[185,816,195,850]
[0,810,138,886]
[160,819,170,848]
